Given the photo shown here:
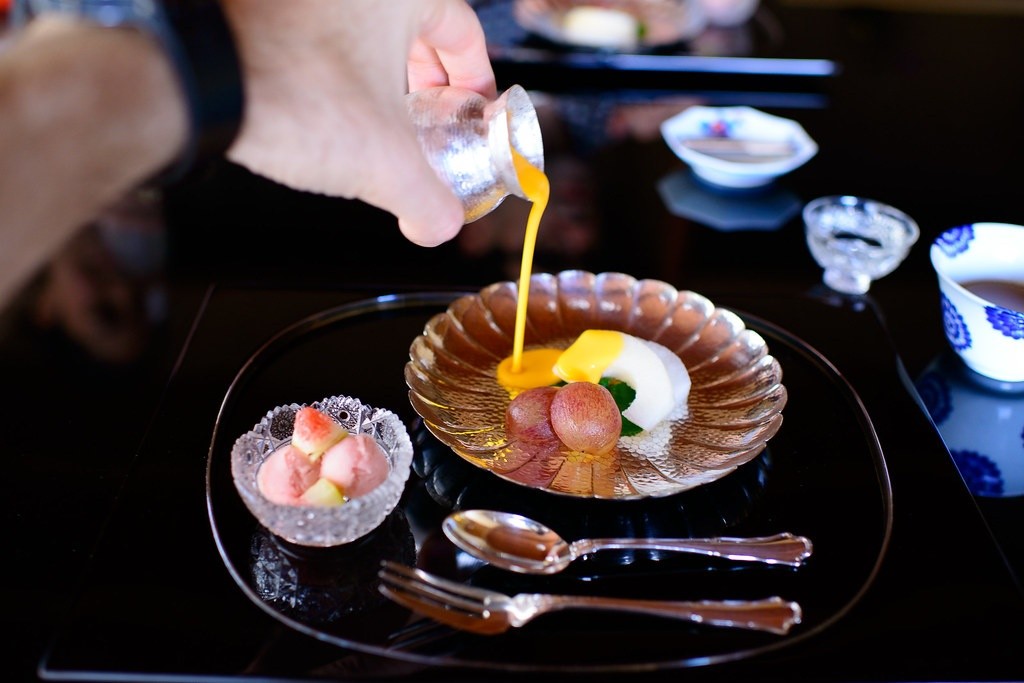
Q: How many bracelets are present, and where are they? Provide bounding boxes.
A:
[78,0,247,174]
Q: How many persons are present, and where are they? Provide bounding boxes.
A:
[0,1,501,309]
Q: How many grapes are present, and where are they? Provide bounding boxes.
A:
[504,382,621,457]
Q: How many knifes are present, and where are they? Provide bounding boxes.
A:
[489,39,832,91]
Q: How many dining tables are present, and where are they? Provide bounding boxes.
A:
[1,0,1024,683]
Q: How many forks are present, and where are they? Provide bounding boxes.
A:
[372,557,802,646]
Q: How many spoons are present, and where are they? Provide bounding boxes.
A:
[439,509,814,579]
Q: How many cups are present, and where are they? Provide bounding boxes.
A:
[412,82,549,229]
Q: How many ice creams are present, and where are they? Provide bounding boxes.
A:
[256,408,390,507]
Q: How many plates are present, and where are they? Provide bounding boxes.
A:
[205,282,893,676]
[403,270,789,505]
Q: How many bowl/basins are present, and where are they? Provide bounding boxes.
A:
[806,198,916,294]
[657,102,817,187]
[226,395,418,547]
[929,220,1023,383]
[923,373,1021,499]
[661,172,799,230]
